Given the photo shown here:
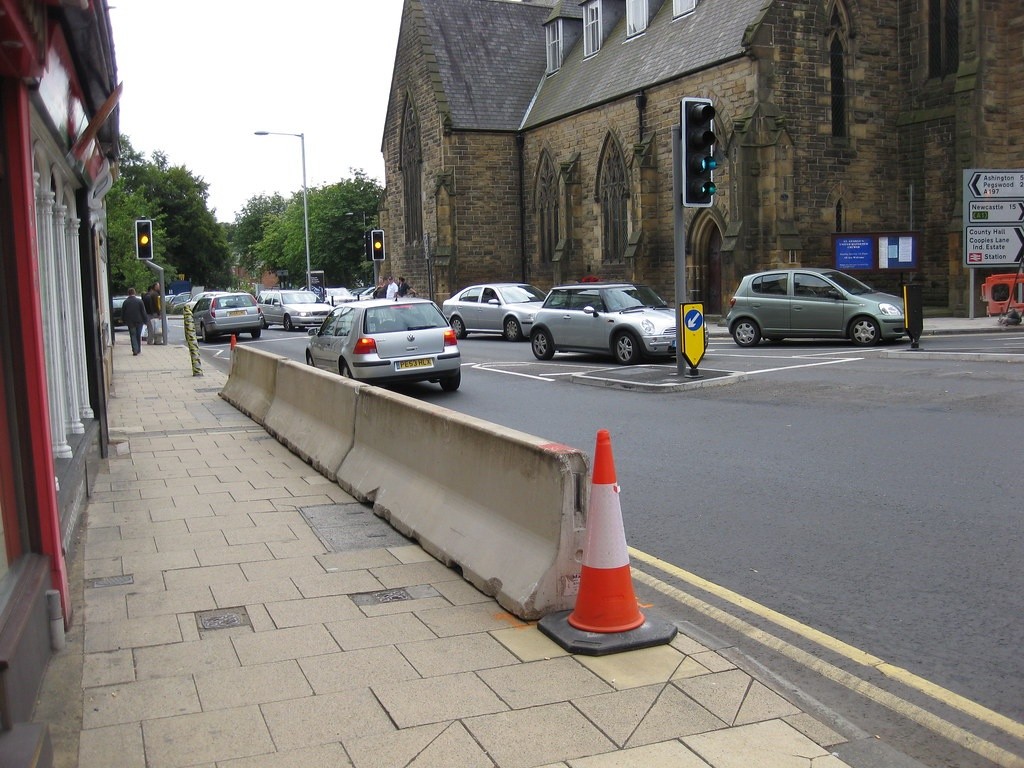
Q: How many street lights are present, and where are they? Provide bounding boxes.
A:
[253,130,312,291]
[344,211,367,231]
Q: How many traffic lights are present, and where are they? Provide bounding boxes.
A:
[363,230,374,262]
[370,230,385,261]
[135,220,154,260]
[680,97,718,209]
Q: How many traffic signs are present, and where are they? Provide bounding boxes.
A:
[967,170,1024,198]
[966,225,1024,265]
[968,201,1024,225]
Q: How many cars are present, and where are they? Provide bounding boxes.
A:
[530,282,710,368]
[191,291,267,345]
[442,283,560,343]
[112,289,229,327]
[305,297,463,392]
[299,284,377,305]
[725,267,909,348]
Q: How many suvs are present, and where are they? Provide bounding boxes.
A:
[256,289,337,332]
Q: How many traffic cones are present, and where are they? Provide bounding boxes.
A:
[537,428,681,657]
[229,334,238,379]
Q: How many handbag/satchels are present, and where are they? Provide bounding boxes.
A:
[151,318,163,335]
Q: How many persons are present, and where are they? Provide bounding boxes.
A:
[374,276,387,299]
[386,275,398,299]
[122,288,149,355]
[403,284,421,298]
[141,282,164,345]
[394,277,409,298]
